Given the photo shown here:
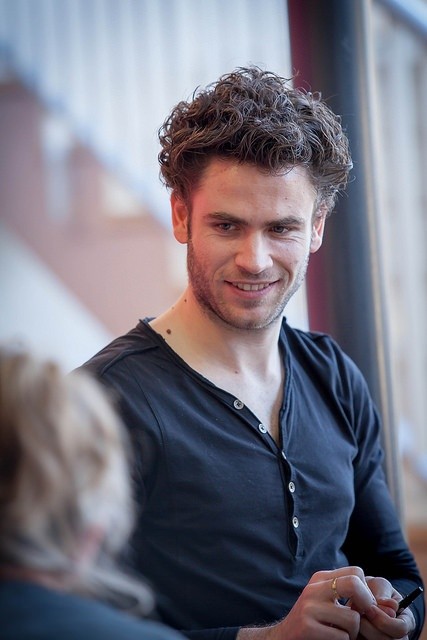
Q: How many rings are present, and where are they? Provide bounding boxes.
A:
[330,576,342,602]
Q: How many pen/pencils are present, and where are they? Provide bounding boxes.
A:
[394,587,424,617]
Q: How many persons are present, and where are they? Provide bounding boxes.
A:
[0,347,186,640]
[64,66,425,640]
